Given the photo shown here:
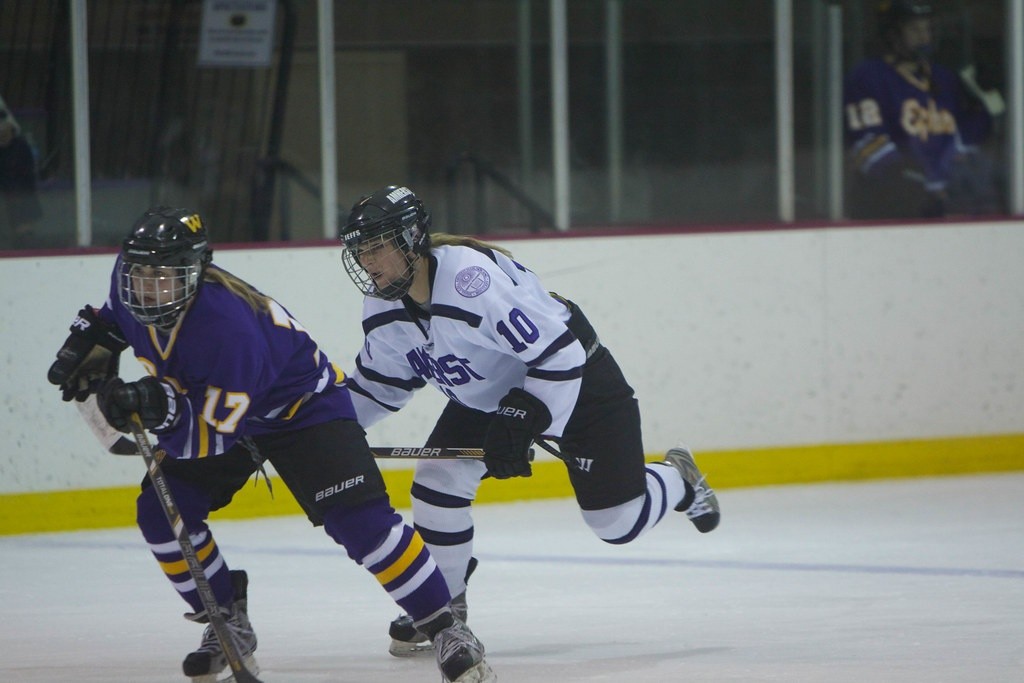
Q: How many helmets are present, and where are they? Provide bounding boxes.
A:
[117,205,213,329]
[337,184,432,302]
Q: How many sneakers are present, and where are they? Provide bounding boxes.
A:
[663,444,721,534]
[412,607,499,683]
[182,569,258,683]
[388,556,478,658]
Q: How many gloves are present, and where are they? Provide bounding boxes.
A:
[46,304,130,403]
[483,387,553,480]
[96,372,188,434]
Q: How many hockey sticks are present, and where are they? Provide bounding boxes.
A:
[75,390,535,462]
[125,406,264,683]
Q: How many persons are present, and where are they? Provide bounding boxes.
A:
[0,98,43,223]
[336,186,720,656]
[47,206,499,683]
[840,0,1009,218]
